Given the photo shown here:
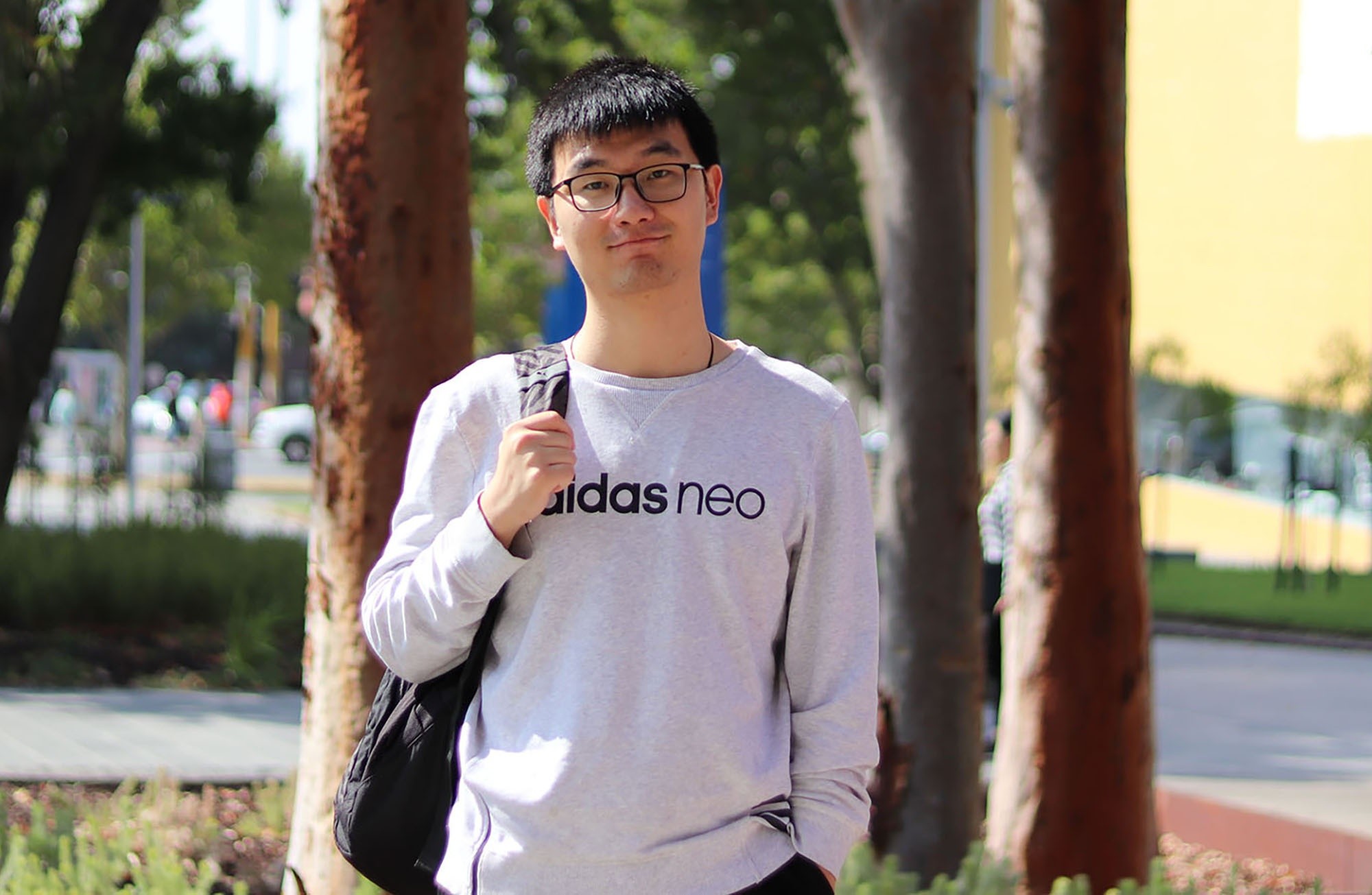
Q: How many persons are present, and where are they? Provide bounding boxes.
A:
[977,406,1011,751]
[361,50,881,895]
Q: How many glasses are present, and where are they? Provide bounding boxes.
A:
[548,162,706,211]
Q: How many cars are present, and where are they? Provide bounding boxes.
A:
[20,346,316,481]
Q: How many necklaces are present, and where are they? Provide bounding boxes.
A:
[706,331,714,369]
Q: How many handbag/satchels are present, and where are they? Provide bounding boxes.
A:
[331,338,573,894]
